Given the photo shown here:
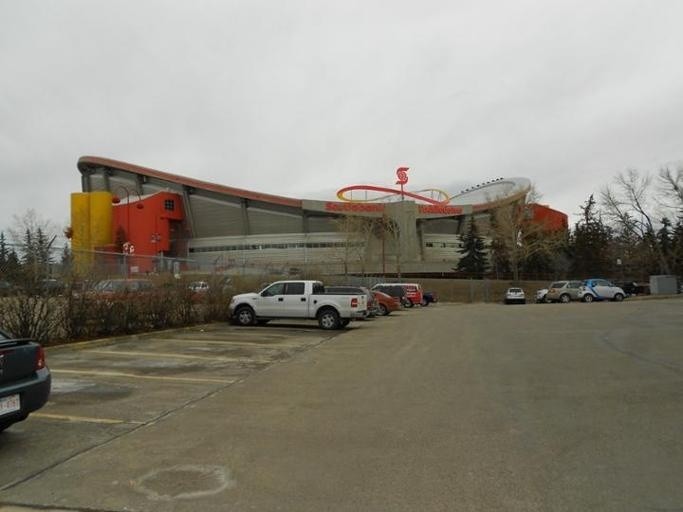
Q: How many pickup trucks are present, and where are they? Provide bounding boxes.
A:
[228,280,368,330]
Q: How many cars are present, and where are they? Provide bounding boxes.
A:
[537,278,651,304]
[1,327,52,429]
[504,288,526,304]
[323,283,440,320]
[93,279,208,295]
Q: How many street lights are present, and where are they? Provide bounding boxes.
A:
[111,185,144,280]
[151,231,160,256]
